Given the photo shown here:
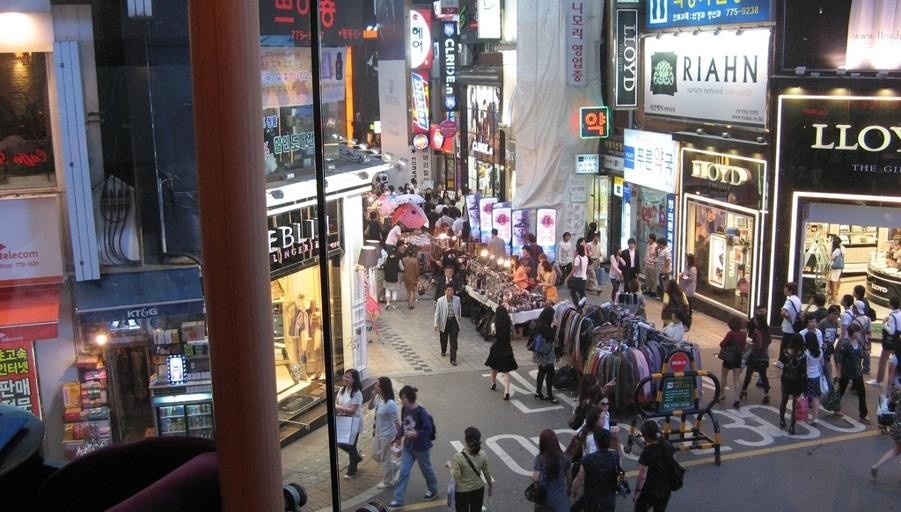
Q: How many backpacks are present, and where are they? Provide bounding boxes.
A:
[789,300,803,333]
[565,436,582,481]
[428,414,436,441]
[781,361,801,388]
[384,263,398,282]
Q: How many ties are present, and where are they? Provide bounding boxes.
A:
[448,278,451,283]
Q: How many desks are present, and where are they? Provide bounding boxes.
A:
[466,281,551,339]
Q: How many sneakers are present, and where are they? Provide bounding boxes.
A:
[756,380,771,389]
[643,289,662,300]
[344,469,357,479]
[773,360,784,369]
[586,288,594,291]
[870,464,880,479]
[862,370,870,375]
[808,420,818,425]
[385,304,390,309]
[424,489,439,502]
[409,305,415,309]
[387,500,404,512]
[357,455,363,463]
[597,289,602,294]
[393,304,399,309]
[833,376,841,381]
[865,379,881,386]
[377,479,394,489]
[393,463,400,477]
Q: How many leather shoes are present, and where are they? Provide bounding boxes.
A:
[789,426,796,434]
[441,351,446,357]
[779,419,786,429]
[860,416,871,423]
[834,411,844,417]
[450,360,458,366]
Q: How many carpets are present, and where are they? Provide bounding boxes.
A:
[276,393,320,416]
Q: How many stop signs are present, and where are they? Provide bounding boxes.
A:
[669,351,690,371]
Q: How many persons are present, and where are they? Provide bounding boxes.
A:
[284,293,306,369]
[334,368,362,480]
[695,208,719,242]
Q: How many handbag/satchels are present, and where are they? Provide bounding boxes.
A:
[752,358,769,369]
[616,466,632,497]
[524,482,544,503]
[831,254,844,269]
[336,415,360,446]
[418,287,425,296]
[446,479,456,508]
[822,388,841,412]
[446,318,459,332]
[795,396,808,421]
[882,336,896,349]
[525,328,554,354]
[567,276,583,289]
[718,345,737,362]
[867,306,876,321]
[669,456,686,491]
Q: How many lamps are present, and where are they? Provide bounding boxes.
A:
[353,239,381,277]
[346,138,407,168]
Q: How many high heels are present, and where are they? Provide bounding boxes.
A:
[504,393,510,401]
[878,424,891,435]
[718,396,725,403]
[534,392,561,404]
[739,391,748,400]
[761,395,771,406]
[733,401,740,408]
[490,383,496,390]
